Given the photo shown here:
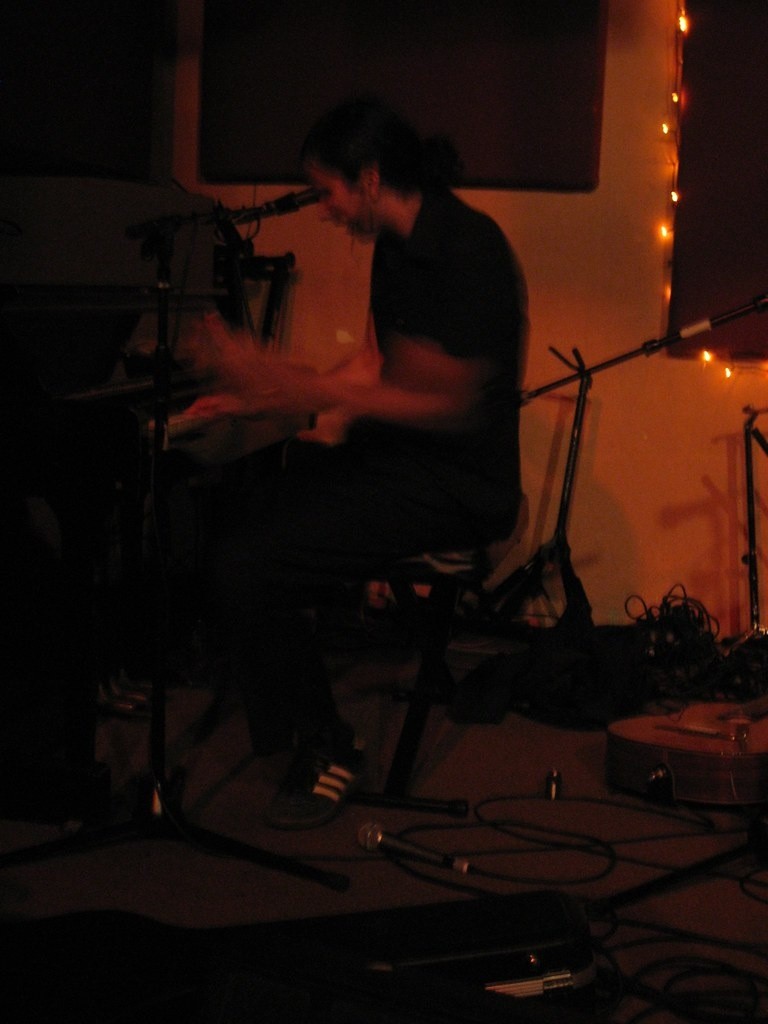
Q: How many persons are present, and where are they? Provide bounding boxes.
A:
[146,99,530,828]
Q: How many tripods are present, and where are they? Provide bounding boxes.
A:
[0,205,351,894]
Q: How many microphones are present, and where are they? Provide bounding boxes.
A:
[233,189,320,228]
[358,823,477,876]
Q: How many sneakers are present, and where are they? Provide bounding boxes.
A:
[270,739,366,832]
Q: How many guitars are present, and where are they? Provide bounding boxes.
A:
[607,694,768,803]
